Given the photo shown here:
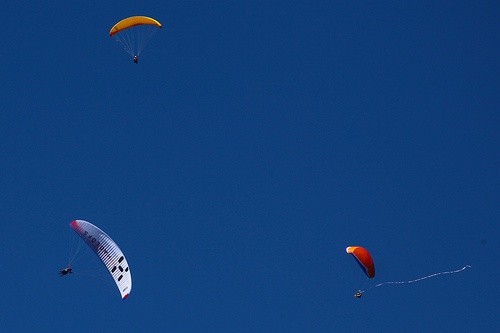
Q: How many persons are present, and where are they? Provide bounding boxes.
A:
[354,289,363,300]
[58,265,72,277]
[133,55,138,64]
